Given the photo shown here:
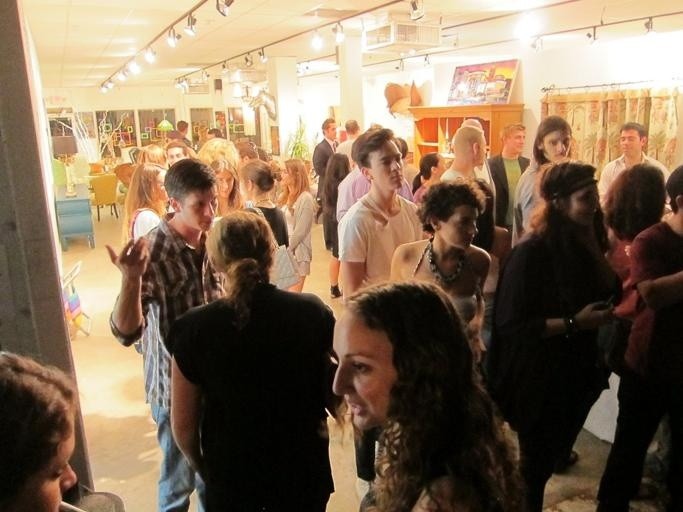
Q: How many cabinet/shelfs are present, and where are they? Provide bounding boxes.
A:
[406,101,523,165]
[55,194,98,252]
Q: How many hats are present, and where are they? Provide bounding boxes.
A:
[539,158,598,202]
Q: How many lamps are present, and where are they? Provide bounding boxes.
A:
[51,136,81,196]
[100,0,234,91]
[529,8,659,54]
[156,109,173,132]
[170,0,425,88]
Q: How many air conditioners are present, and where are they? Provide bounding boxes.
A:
[359,11,443,56]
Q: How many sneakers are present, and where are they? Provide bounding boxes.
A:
[553,451,578,475]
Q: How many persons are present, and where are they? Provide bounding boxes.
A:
[332,279,530,511]
[118,110,682,477]
[336,124,425,498]
[103,158,231,512]
[489,161,633,511]
[169,210,335,511]
[593,164,682,511]
[0,349,90,512]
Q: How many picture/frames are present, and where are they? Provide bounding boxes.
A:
[269,124,280,156]
[231,123,243,133]
[96,119,206,148]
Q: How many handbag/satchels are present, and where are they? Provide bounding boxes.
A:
[269,244,303,290]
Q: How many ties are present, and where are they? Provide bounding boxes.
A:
[333,142,336,153]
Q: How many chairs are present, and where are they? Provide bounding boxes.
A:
[51,160,75,194]
[86,172,118,218]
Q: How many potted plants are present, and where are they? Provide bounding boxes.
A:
[286,128,312,176]
